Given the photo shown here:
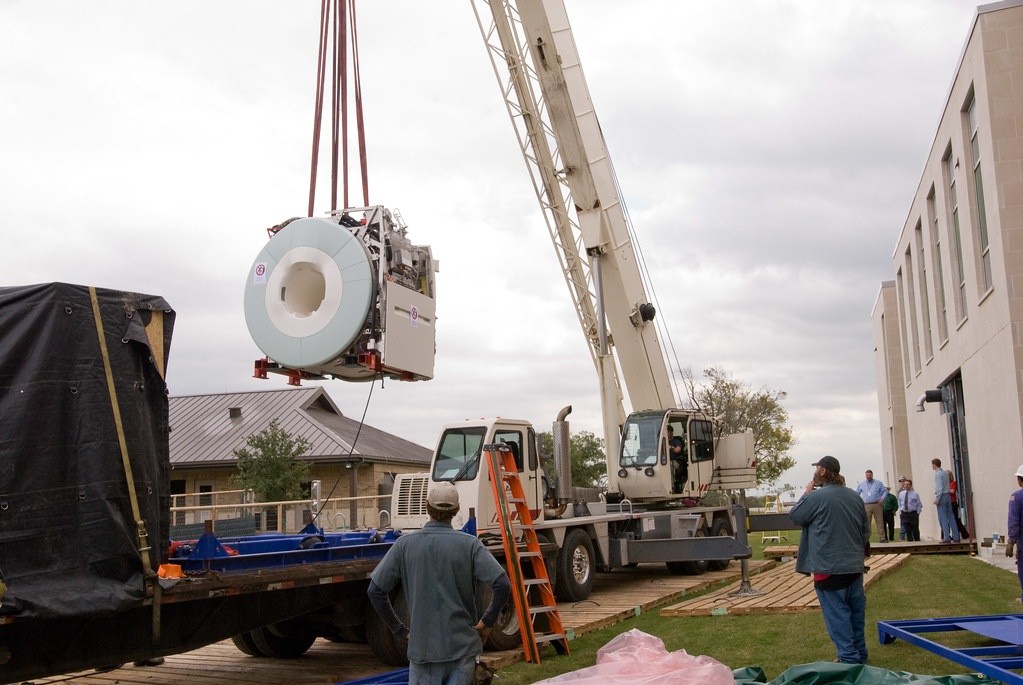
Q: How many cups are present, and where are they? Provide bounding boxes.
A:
[993,534,998,543]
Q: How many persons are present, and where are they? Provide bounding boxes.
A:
[896,476,914,540]
[96,657,164,671]
[667,425,682,460]
[367,481,511,685]
[940,470,970,540]
[883,484,898,541]
[1006,465,1023,602]
[898,479,922,541]
[789,456,869,665]
[856,470,888,542]
[932,458,960,543]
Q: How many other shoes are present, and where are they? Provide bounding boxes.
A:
[832,658,844,663]
[880,539,888,543]
[951,541,960,544]
[939,541,950,544]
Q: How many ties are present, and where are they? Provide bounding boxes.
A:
[905,492,908,510]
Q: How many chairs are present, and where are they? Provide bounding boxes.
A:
[504,440,520,469]
[673,437,686,482]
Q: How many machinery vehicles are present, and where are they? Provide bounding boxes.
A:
[390,0,756,601]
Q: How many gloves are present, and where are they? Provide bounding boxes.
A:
[1005,541,1013,557]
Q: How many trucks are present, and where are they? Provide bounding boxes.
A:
[0,278,483,683]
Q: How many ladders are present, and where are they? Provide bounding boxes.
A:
[761,494,790,546]
[482,442,570,663]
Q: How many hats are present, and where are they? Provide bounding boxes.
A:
[885,486,891,490]
[812,456,840,472]
[1014,465,1023,476]
[898,476,906,482]
[427,482,459,510]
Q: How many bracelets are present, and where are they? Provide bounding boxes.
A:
[671,445,672,448]
